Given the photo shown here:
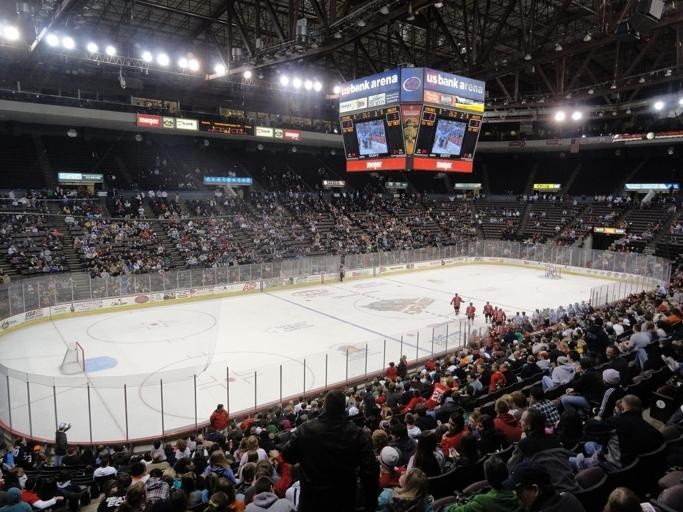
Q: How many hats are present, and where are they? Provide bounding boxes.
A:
[503,460,552,486]
[376,446,400,469]
[603,368,620,384]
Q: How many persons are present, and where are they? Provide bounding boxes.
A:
[1,155,682,512]
